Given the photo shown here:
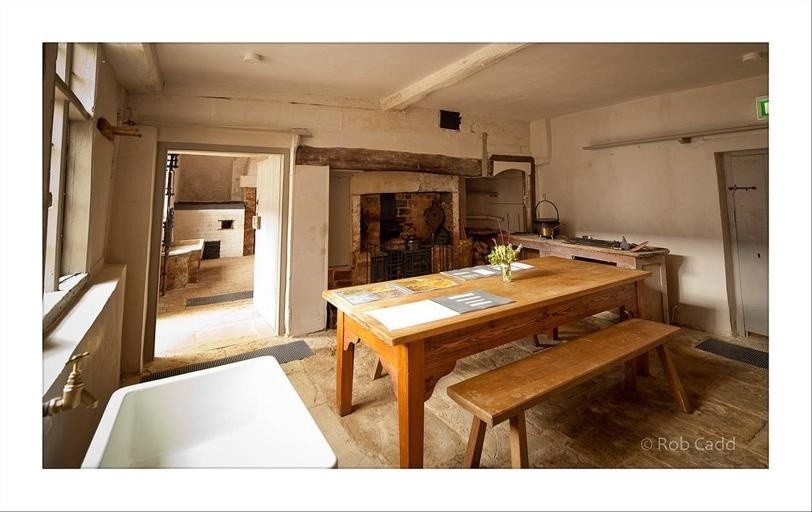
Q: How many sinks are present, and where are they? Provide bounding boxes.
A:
[77,355,338,469]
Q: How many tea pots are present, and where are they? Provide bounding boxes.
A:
[402,227,418,251]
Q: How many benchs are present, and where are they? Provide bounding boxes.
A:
[447,317,694,469]
[369,332,541,379]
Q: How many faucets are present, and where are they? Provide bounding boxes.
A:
[43,352,99,417]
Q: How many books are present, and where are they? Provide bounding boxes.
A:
[430,290,518,313]
[332,282,410,306]
[482,261,536,274]
[363,299,461,332]
[386,273,464,295]
[440,265,501,282]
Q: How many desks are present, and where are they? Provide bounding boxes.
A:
[498,232,670,325]
[321,256,653,469]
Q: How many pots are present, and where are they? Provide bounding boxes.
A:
[532,200,560,237]
